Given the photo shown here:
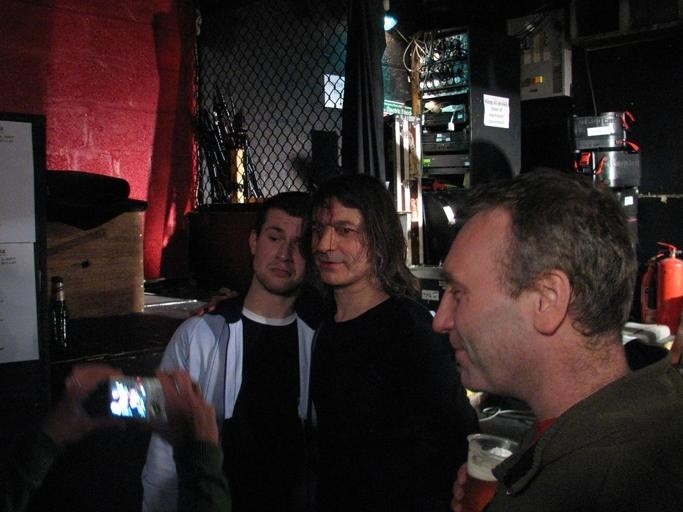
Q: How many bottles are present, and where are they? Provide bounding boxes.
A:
[50,276,70,351]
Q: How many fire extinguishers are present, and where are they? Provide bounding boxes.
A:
[640,242,683,335]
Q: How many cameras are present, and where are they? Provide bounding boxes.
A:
[106,376,189,423]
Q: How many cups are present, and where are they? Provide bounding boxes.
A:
[465,433,520,511]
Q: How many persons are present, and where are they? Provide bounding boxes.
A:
[193,172,480,512]
[430,162,683,511]
[140,190,319,512]
[0,362,234,512]
